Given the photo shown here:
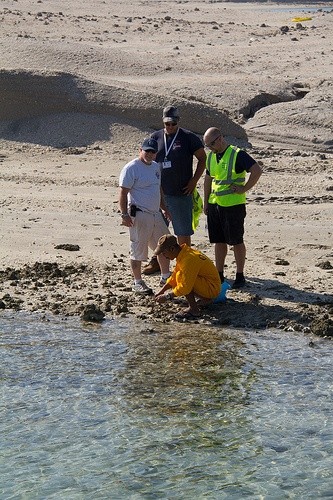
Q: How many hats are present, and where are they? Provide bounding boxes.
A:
[153,233,178,255]
[162,106,181,122]
[140,136,159,152]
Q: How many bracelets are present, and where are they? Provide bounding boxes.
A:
[121,213,129,218]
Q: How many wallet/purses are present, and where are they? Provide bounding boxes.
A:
[130,204,137,216]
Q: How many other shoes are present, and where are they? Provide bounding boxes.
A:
[142,255,160,274]
[220,272,225,283]
[196,300,214,308]
[175,308,202,320]
[232,276,245,288]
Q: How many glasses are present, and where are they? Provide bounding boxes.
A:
[141,149,157,154]
[162,122,179,126]
[203,135,221,148]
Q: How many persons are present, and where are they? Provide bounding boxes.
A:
[143,106,207,274]
[203,127,262,288]
[154,234,221,317]
[118,138,173,294]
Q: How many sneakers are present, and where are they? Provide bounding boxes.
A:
[133,282,153,295]
[159,275,171,287]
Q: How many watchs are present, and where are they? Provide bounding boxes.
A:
[166,292,172,300]
